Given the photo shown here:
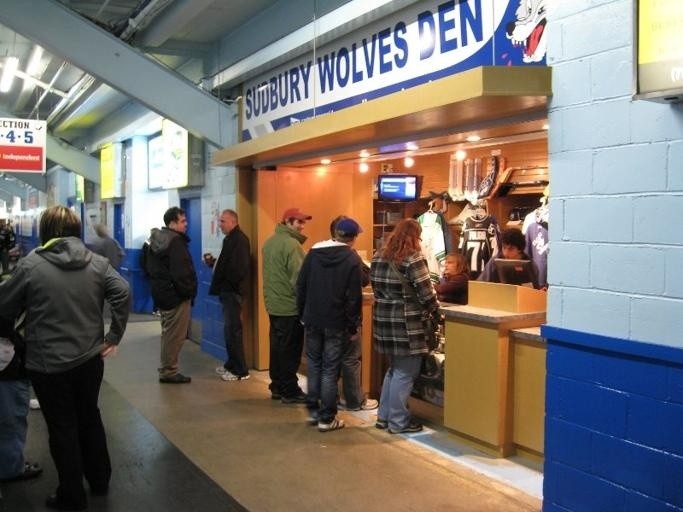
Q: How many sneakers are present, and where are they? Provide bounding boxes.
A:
[305,412,345,431]
[46,493,87,512]
[216,367,250,381]
[376,419,422,433]
[271,391,307,403]
[338,397,378,411]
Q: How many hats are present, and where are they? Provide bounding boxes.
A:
[282,208,311,223]
[336,219,364,237]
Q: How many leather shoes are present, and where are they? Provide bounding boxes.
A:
[160,373,191,383]
[0,462,45,480]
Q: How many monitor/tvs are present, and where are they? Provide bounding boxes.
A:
[495,259,539,289]
[379,174,417,201]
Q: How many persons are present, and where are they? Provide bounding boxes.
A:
[431,250,472,303]
[204,208,254,381]
[368,218,446,434]
[1,218,43,485]
[294,218,365,432]
[474,228,539,289]
[0,204,131,512]
[93,223,124,319]
[261,208,310,403]
[330,213,377,410]
[139,207,198,384]
[141,226,165,317]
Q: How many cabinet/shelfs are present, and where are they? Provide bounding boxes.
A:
[373,198,429,257]
